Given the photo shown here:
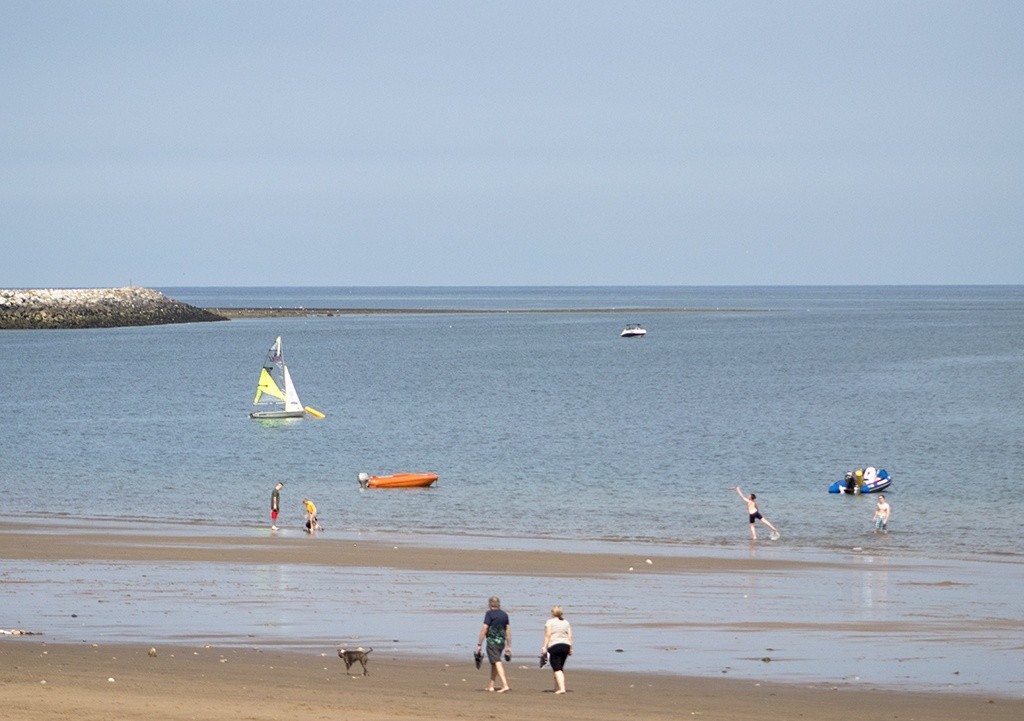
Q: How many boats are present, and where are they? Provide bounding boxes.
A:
[359,472,439,490]
[620,323,647,337]
[829,466,892,493]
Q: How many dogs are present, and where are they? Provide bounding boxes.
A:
[339,647,373,676]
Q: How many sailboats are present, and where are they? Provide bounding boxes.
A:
[249,335,306,417]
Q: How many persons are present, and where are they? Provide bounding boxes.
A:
[735,484,777,541]
[476,595,513,693]
[270,481,284,531]
[302,498,322,536]
[540,604,574,695]
[871,494,891,536]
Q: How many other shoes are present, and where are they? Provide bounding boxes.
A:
[540,654,547,667]
[474,650,484,669]
[272,527,279,530]
